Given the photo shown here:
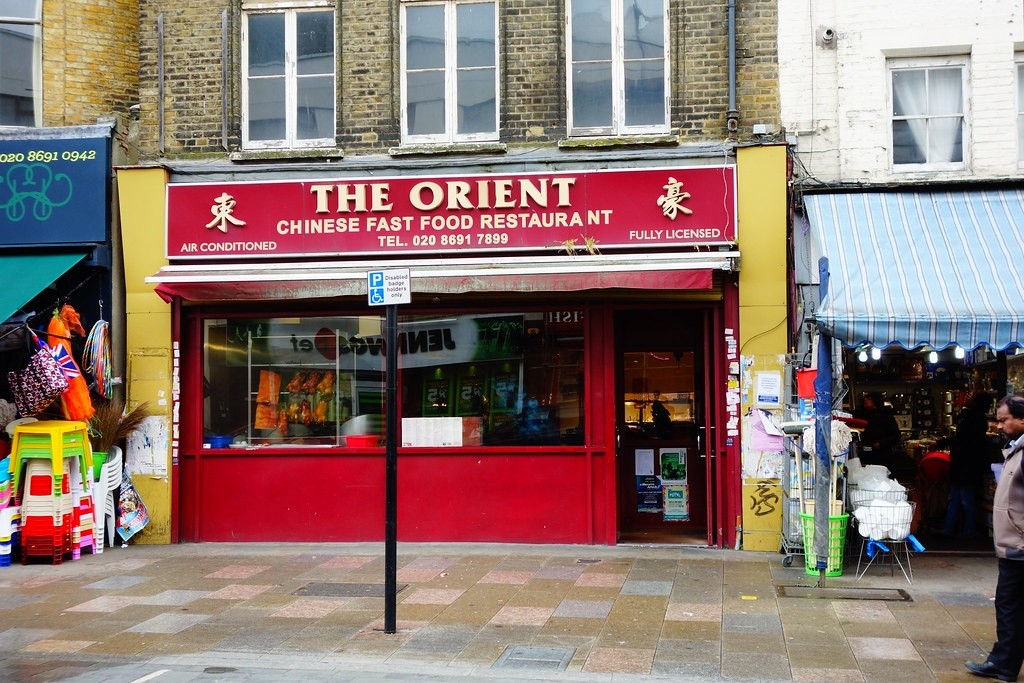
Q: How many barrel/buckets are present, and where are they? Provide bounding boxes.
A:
[91,452,109,479]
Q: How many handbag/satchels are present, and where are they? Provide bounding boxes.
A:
[7,330,69,418]
[46,343,81,380]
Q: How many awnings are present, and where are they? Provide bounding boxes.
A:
[804,186,1024,350]
[0,252,89,339]
[146,251,742,304]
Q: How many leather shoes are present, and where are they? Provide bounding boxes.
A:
[965,660,1018,682]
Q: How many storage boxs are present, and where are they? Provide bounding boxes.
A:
[893,413,914,431]
[635,446,693,523]
[783,402,854,544]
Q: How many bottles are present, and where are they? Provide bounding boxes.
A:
[422,363,518,417]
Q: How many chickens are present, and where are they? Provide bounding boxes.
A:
[277,398,329,436]
[284,368,336,396]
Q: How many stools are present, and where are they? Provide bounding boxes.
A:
[0,419,96,568]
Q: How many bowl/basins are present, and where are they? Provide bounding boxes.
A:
[205,434,233,448]
[343,435,381,448]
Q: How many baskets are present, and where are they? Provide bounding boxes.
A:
[798,512,849,577]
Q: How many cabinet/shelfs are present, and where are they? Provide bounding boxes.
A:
[247,328,345,447]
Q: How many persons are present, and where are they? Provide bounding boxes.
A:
[862,388,1024,683]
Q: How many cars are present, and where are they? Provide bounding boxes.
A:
[483,400,649,456]
[230,416,336,446]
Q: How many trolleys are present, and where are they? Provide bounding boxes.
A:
[778,434,848,567]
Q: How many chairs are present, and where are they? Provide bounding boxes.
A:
[96,445,123,554]
